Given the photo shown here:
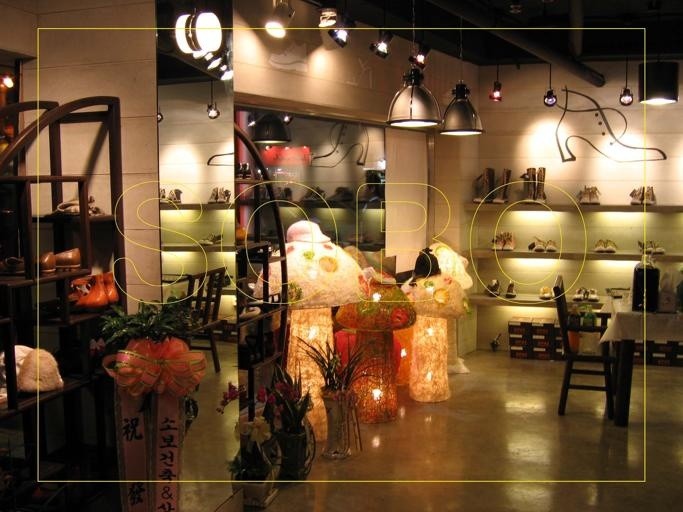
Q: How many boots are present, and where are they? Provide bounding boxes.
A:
[471,166,495,203]
[492,168,512,204]
[533,168,546,204]
[520,168,537,204]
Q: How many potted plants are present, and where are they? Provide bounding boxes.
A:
[85,287,215,512]
[290,327,374,459]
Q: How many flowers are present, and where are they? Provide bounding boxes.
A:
[224,410,288,485]
[250,350,315,428]
[213,381,240,418]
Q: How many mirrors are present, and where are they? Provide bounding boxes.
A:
[153,4,245,512]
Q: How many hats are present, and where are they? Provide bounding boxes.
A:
[414,247,440,275]
[0,343,35,382]
[16,348,65,394]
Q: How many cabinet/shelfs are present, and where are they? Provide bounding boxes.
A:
[0,96,127,511]
[464,200,682,314]
[160,200,233,299]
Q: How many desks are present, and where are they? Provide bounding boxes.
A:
[592,291,682,429]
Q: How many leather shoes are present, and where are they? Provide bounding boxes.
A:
[206,274,234,290]
[53,246,82,273]
[33,269,121,316]
[0,250,58,277]
[238,344,261,367]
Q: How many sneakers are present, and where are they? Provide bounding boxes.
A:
[533,236,559,253]
[487,278,518,299]
[197,232,224,247]
[207,186,232,204]
[630,185,658,207]
[159,186,182,205]
[539,285,601,302]
[489,230,518,251]
[638,238,668,256]
[578,185,601,207]
[235,162,387,204]
[593,237,619,254]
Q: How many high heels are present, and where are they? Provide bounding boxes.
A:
[238,288,249,307]
[235,224,248,246]
[240,385,247,402]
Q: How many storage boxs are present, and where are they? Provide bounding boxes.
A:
[503,317,682,367]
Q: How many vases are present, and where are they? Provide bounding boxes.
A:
[270,424,319,484]
[229,466,281,510]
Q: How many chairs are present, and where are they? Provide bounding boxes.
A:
[178,264,228,393]
[545,275,619,420]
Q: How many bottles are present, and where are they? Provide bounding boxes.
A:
[633,250,659,315]
[567,302,598,332]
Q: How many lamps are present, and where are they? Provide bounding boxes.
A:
[153,1,235,123]
